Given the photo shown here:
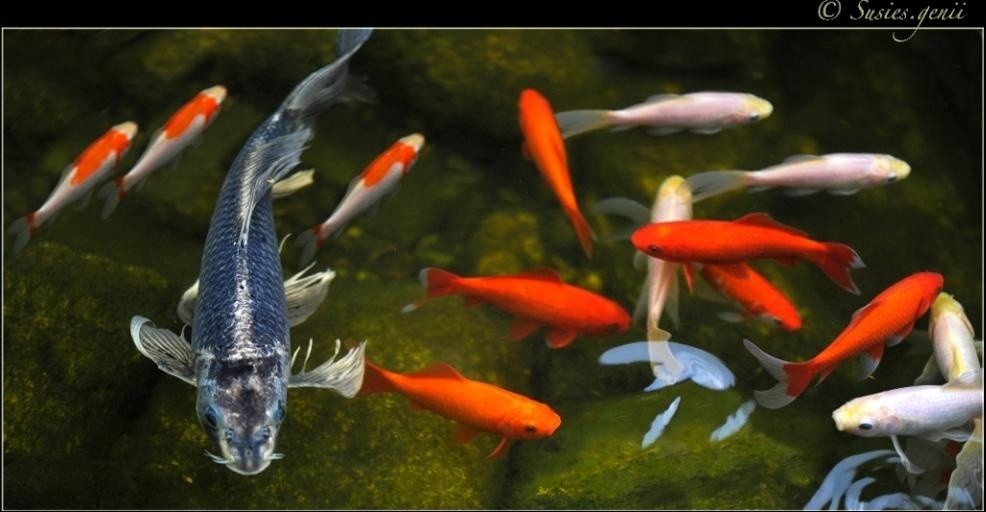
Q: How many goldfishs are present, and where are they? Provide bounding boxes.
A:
[635,150,981,477]
